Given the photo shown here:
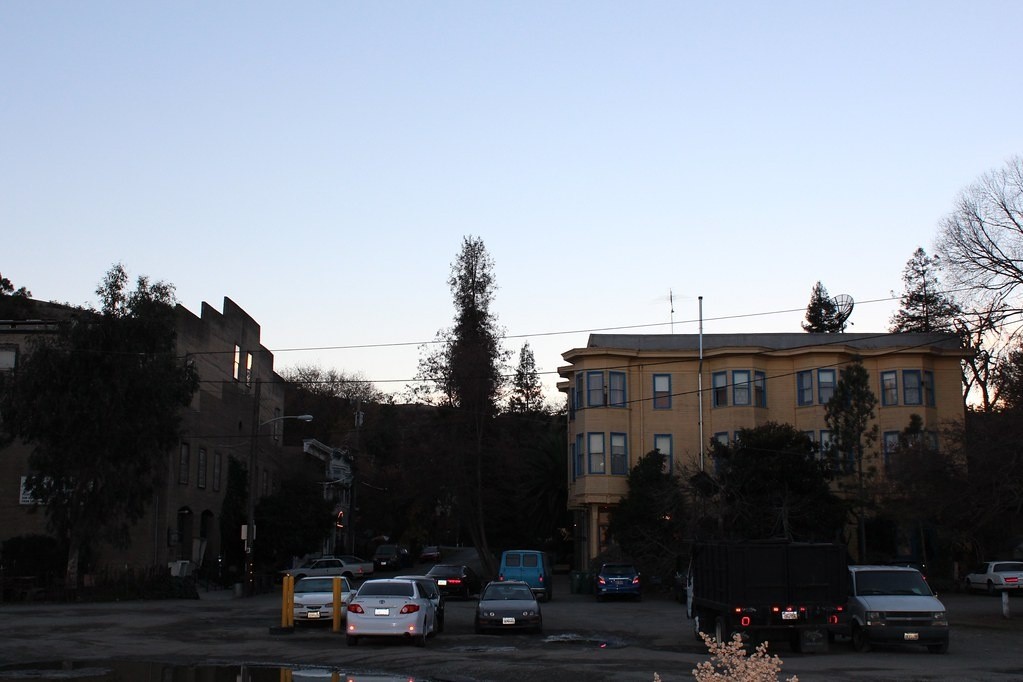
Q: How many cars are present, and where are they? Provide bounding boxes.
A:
[596,562,641,602]
[962,562,1023,597]
[316,556,374,578]
[293,576,357,627]
[278,558,364,582]
[345,575,444,646]
[425,564,480,600]
[474,578,543,635]
[419,546,442,564]
[372,544,411,571]
[828,564,949,654]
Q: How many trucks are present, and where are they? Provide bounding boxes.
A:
[685,542,844,654]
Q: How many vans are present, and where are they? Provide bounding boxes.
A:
[499,550,553,601]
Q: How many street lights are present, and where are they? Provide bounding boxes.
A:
[246,415,313,598]
